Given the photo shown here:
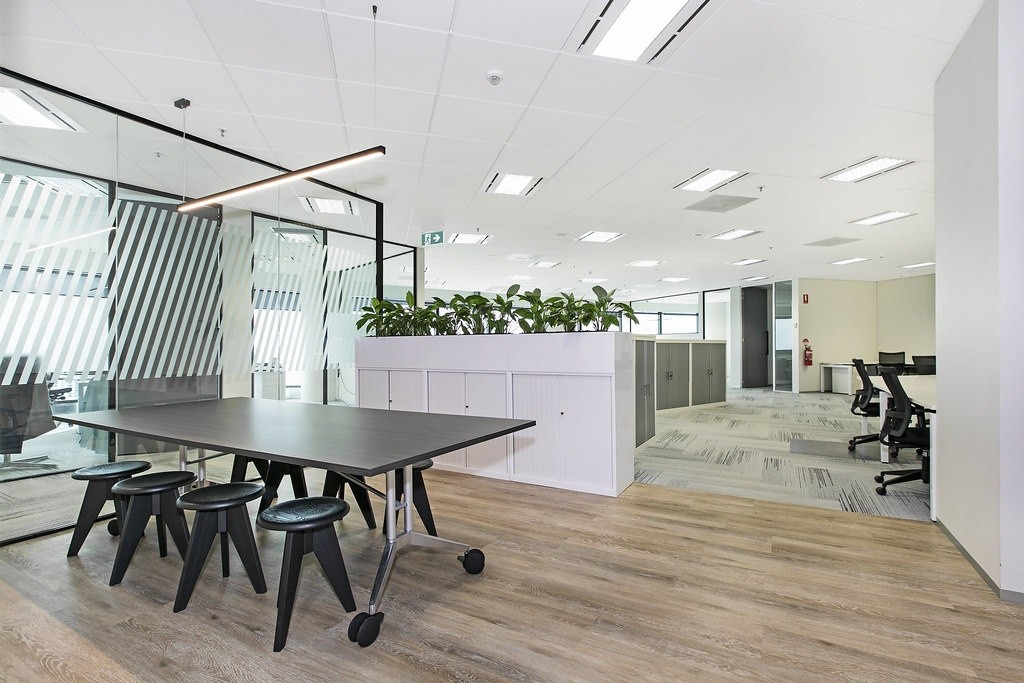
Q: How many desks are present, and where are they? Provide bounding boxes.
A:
[51,396,537,647]
[820,362,916,395]
[856,374,937,522]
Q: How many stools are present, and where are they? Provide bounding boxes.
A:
[257,496,357,653]
[67,461,153,557]
[173,482,268,613]
[108,471,199,587]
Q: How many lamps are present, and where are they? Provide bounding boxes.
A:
[174,5,386,212]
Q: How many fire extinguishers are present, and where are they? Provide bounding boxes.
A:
[804,343,814,367]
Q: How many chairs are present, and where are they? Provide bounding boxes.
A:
[0,356,57,470]
[878,351,905,375]
[912,355,936,375]
[874,366,936,495]
[848,358,924,457]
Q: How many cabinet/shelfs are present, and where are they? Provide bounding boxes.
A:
[354,331,635,497]
[655,339,692,414]
[691,339,728,408]
[633,333,657,456]
